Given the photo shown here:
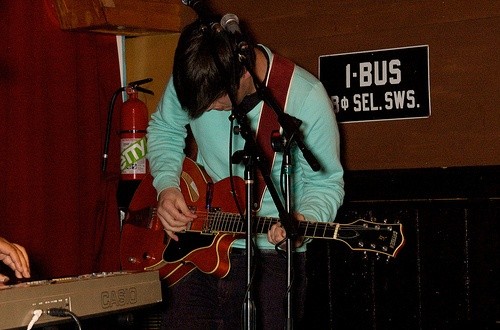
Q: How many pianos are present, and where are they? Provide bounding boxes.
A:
[0,270,162,330]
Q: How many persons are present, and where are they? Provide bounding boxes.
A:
[146,14,345,330]
[0,237,31,283]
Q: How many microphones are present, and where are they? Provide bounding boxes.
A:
[182,0,222,33]
[221,13,248,51]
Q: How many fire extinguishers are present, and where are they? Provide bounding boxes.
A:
[101,78,156,183]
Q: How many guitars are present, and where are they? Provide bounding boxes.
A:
[119,156,405,282]
